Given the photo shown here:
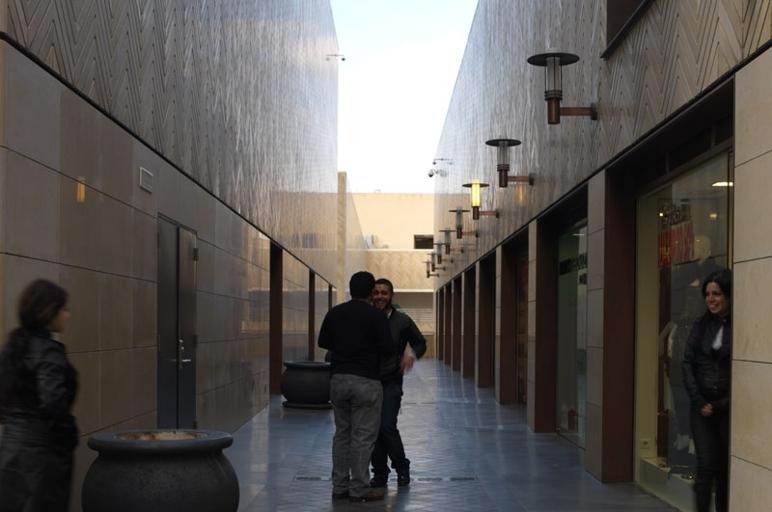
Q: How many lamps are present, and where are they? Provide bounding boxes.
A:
[423,139,534,278]
[527,53,598,123]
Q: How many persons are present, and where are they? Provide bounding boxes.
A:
[317,269,401,502]
[685,267,729,510]
[0,279,80,510]
[370,278,426,486]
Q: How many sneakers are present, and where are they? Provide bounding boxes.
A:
[331,457,411,504]
